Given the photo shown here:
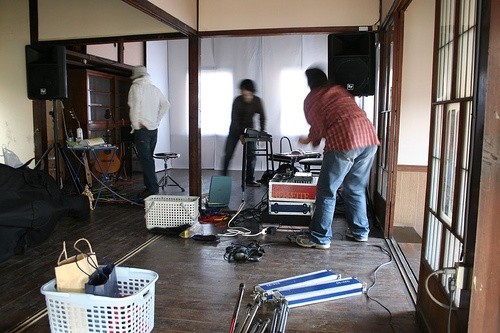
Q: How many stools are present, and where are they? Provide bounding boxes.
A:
[154,153,185,191]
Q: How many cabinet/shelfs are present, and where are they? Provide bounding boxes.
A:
[63,70,131,180]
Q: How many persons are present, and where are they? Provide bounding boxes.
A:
[127,63,170,202]
[294,68,380,249]
[219,80,267,187]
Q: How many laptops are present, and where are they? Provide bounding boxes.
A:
[205,175,232,208]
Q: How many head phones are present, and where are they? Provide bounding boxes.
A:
[233,246,264,262]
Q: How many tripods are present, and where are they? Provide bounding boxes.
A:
[32,101,84,196]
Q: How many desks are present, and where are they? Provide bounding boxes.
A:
[242,137,274,191]
[67,144,118,209]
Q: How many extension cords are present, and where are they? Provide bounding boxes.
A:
[262,226,269,235]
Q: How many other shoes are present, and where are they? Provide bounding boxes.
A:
[246,180,261,187]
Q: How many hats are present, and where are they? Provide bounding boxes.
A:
[130,65,148,79]
[240,79,255,92]
[305,68,327,86]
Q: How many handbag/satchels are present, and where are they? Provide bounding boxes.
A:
[76,252,119,297]
[55,238,98,291]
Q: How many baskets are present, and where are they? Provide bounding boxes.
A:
[41,265,159,333]
[144,194,199,230]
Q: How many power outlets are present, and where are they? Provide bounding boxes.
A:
[454,263,465,306]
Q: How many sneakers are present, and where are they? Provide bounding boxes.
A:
[346,228,368,242]
[296,234,331,249]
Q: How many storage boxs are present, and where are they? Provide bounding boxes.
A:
[144,195,200,229]
[269,202,313,215]
[269,173,319,202]
[258,269,367,310]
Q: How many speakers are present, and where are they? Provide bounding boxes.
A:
[327,31,376,96]
[25,43,68,100]
